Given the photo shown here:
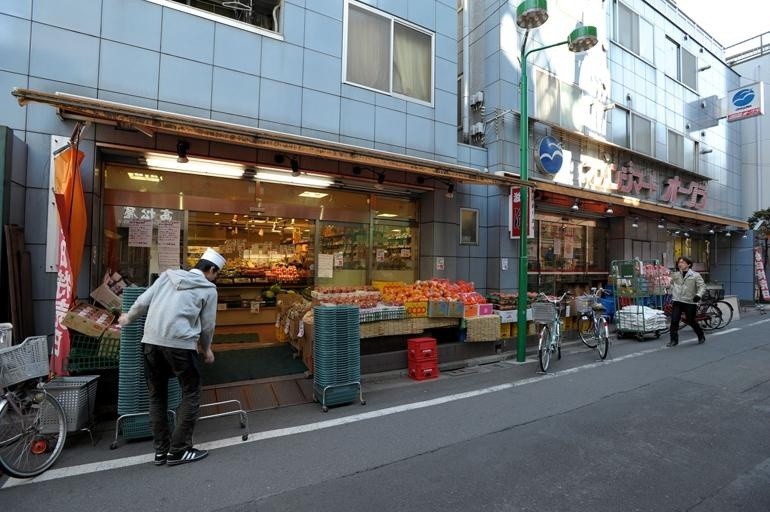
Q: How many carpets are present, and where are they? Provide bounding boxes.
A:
[197,333,307,387]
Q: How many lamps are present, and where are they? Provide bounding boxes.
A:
[351,165,386,190]
[416,176,454,199]
[570,198,582,211]
[175,140,191,163]
[605,202,614,215]
[275,154,302,177]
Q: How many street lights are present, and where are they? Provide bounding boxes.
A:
[517,0,599,362]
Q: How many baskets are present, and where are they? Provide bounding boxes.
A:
[40,375,100,434]
[702,288,724,301]
[314,305,361,406]
[118,288,182,440]
[360,308,382,322]
[600,286,673,316]
[63,335,117,371]
[383,306,406,320]
[575,295,594,312]
[500,317,590,339]
[531,303,557,324]
[0,335,49,388]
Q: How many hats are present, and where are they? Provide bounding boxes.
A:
[200,247,226,271]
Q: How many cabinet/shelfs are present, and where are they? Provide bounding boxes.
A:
[214,285,310,327]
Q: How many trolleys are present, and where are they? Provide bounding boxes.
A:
[612,257,664,342]
[30,370,101,455]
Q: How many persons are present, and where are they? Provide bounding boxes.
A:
[664,256,706,347]
[117,246,226,468]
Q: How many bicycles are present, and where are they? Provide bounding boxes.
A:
[0,322,68,478]
[665,285,734,328]
[664,301,723,329]
[532,290,572,372]
[574,288,618,359]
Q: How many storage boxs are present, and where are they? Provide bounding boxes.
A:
[405,302,590,339]
[59,271,139,359]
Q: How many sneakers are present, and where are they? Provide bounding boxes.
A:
[698,336,705,344]
[666,341,678,346]
[154,454,167,465]
[167,448,208,466]
